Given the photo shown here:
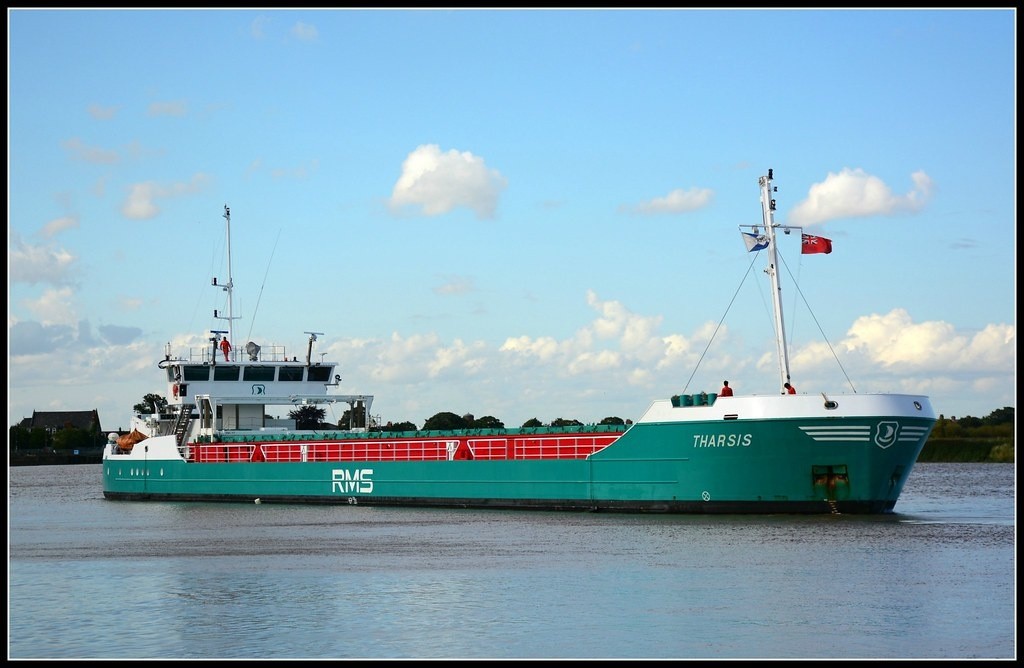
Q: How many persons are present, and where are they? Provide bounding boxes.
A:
[784,383,796,394]
[220,337,232,362]
[717,380,733,397]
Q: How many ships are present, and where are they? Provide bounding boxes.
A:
[102,166,935,518]
